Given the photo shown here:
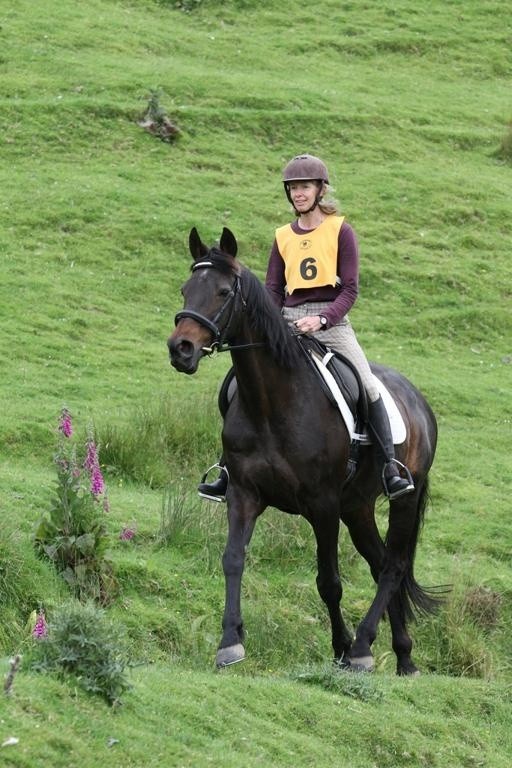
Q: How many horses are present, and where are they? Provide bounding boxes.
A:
[168,228,454,681]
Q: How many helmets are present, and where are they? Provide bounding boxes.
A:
[283,155,328,182]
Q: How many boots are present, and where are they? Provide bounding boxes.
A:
[360,394,410,494]
[198,455,226,496]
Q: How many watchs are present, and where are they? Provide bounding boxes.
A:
[319,315,328,327]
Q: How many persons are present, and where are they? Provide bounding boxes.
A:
[197,152,412,498]
